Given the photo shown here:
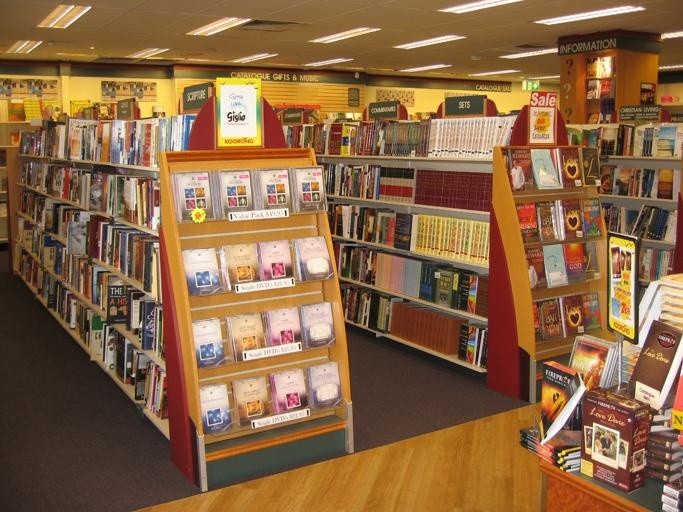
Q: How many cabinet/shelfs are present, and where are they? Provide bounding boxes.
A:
[568,142,683,303]
[585,49,617,125]
[4,148,355,494]
[0,74,62,243]
[315,147,607,404]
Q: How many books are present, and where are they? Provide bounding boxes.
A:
[0,98,342,440]
[505,123,683,512]
[280,114,516,369]
[584,55,617,99]
[519,438,683,511]
[639,82,656,106]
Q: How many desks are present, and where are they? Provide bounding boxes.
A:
[538,457,683,511]
